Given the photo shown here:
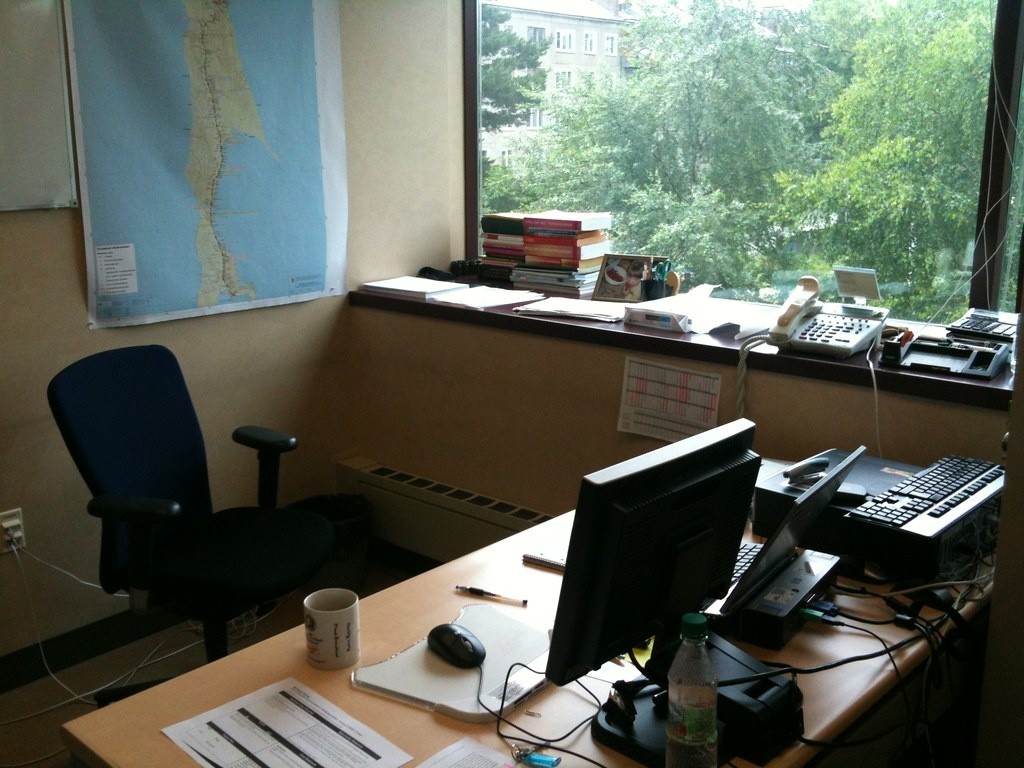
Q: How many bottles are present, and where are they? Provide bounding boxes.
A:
[666,613,717,768]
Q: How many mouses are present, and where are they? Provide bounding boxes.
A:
[430,622,484,666]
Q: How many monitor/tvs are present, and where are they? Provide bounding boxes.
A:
[546,418,763,686]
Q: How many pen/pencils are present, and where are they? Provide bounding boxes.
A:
[642,270,661,281]
[455,584,527,609]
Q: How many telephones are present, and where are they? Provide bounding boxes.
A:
[766,274,893,359]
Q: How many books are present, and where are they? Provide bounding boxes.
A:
[360,208,779,340]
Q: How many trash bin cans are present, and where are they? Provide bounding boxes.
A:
[276,489,382,594]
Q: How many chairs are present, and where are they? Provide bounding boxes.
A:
[47,344,375,709]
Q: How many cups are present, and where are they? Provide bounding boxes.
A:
[303,589,360,670]
[640,279,673,302]
[622,277,641,296]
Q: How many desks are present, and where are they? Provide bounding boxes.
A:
[60,487,999,768]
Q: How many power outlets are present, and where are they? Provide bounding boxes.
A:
[0,507,26,555]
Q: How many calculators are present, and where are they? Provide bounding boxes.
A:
[945,307,1021,343]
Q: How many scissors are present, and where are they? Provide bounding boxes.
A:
[655,259,672,281]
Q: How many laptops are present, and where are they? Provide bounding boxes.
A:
[703,444,866,618]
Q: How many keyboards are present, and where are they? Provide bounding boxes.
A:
[844,454,1004,539]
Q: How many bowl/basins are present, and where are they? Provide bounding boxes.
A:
[605,264,628,285]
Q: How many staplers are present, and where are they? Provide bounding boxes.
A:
[782,457,829,484]
